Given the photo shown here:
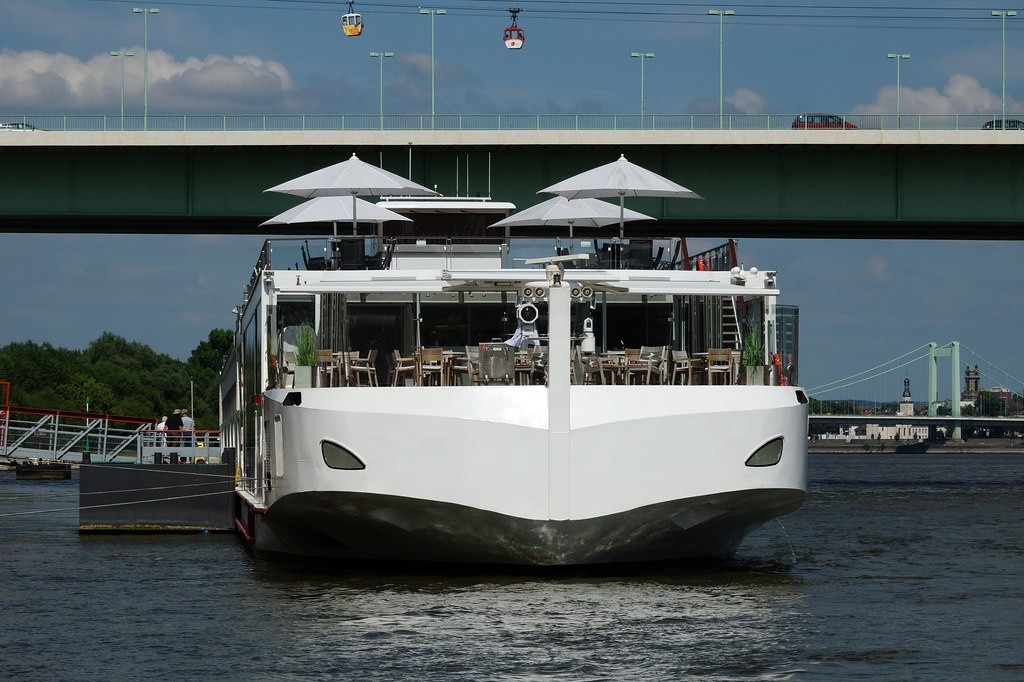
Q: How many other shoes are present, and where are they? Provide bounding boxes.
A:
[180,460,186,464]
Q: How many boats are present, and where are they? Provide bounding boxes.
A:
[808,431,943,455]
[14,458,72,483]
[214,150,812,583]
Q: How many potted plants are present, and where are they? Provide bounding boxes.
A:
[741,318,766,386]
[294,322,316,388]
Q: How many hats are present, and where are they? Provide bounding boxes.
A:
[181,409,188,414]
[162,416,168,421]
[173,409,180,414]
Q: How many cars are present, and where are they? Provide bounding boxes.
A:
[791,111,859,130]
[983,119,1024,130]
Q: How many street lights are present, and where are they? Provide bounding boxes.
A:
[887,53,911,131]
[991,9,1018,130]
[708,9,735,130]
[630,52,655,130]
[419,9,447,130]
[132,7,161,131]
[110,51,136,131]
[369,51,395,131]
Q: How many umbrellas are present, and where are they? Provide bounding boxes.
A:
[263,153,443,236]
[536,153,704,244]
[258,196,413,236]
[487,196,658,255]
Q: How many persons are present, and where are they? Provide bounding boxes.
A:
[180,408,192,464]
[158,416,168,447]
[162,409,183,464]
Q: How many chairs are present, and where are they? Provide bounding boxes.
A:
[672,347,739,385]
[582,345,671,385]
[587,237,680,270]
[388,344,550,387]
[315,349,379,387]
[298,240,395,271]
